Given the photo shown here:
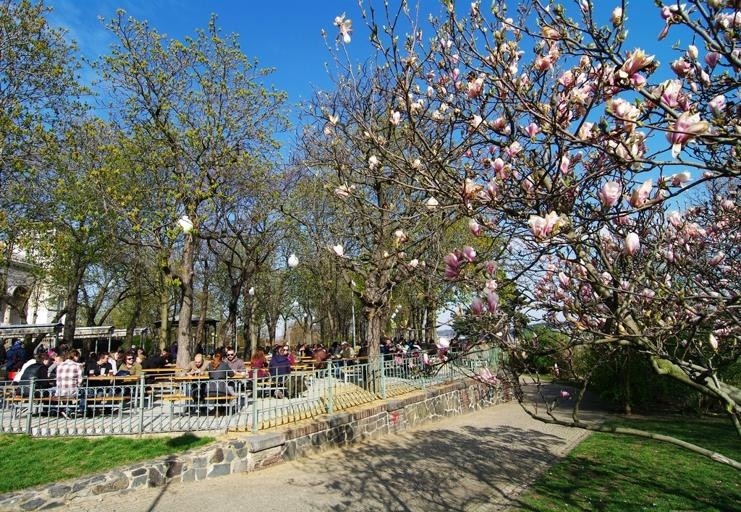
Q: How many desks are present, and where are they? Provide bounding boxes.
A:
[0,356,315,421]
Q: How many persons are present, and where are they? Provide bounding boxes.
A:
[380,335,439,379]
[296,339,368,381]
[448,337,468,352]
[186,342,295,417]
[1,337,178,420]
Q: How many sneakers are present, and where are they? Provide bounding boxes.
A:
[61,409,73,420]
[82,412,93,420]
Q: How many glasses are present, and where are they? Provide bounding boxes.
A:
[127,357,135,360]
[284,349,288,351]
[227,354,234,356]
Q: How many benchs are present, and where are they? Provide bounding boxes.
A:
[0,356,315,421]
